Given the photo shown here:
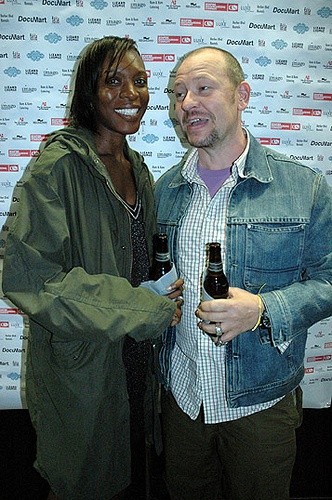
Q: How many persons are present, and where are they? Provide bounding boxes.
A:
[0,36,184,500]
[153,46,332,500]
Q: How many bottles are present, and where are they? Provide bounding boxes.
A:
[150,233,182,323]
[200,243,229,338]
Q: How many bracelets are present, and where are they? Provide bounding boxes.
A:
[252,295,262,332]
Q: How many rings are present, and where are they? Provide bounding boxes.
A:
[215,322,223,337]
[218,338,228,345]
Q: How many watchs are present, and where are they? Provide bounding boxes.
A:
[261,311,271,330]
